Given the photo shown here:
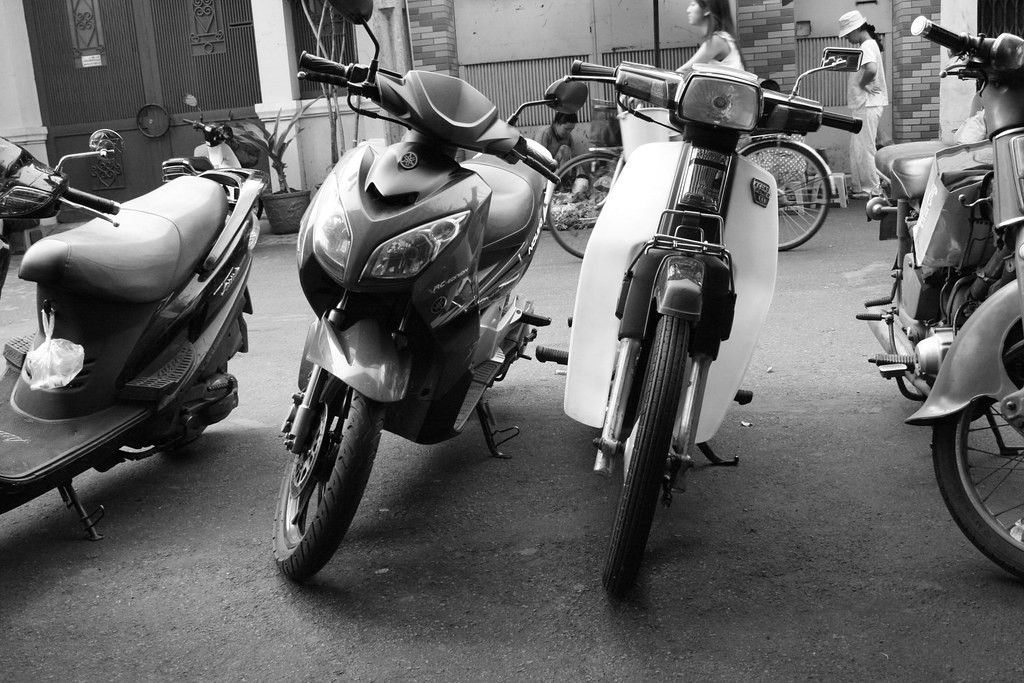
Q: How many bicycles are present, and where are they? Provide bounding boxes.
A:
[546,87,836,261]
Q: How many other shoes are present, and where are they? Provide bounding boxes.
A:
[853,192,869,198]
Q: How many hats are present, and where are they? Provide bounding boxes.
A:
[838,10,866,38]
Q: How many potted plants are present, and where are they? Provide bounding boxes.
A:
[229,93,326,235]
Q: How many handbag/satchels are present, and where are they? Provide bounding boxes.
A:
[28,308,84,390]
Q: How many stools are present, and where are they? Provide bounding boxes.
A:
[8,225,47,256]
[778,180,807,216]
[810,173,849,208]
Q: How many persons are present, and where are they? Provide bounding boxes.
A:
[532,113,592,196]
[629,0,746,107]
[839,11,888,199]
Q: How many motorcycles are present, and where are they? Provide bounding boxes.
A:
[861,15,1023,579]
[269,0,591,580]
[561,46,863,596]
[0,94,271,544]
